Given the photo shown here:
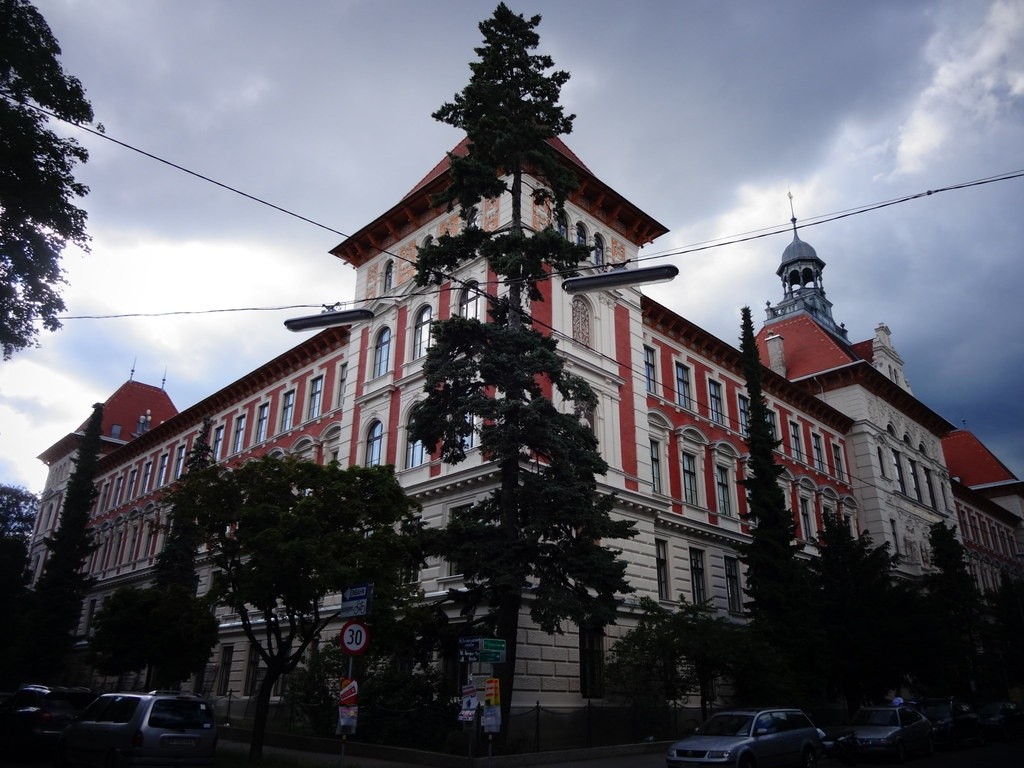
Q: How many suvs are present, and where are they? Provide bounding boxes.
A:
[0,682,102,768]
[888,692,989,753]
[54,689,221,768]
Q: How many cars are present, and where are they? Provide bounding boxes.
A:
[977,699,1024,743]
[841,703,936,765]
[665,705,826,768]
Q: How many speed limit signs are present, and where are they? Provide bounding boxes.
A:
[340,618,371,656]
